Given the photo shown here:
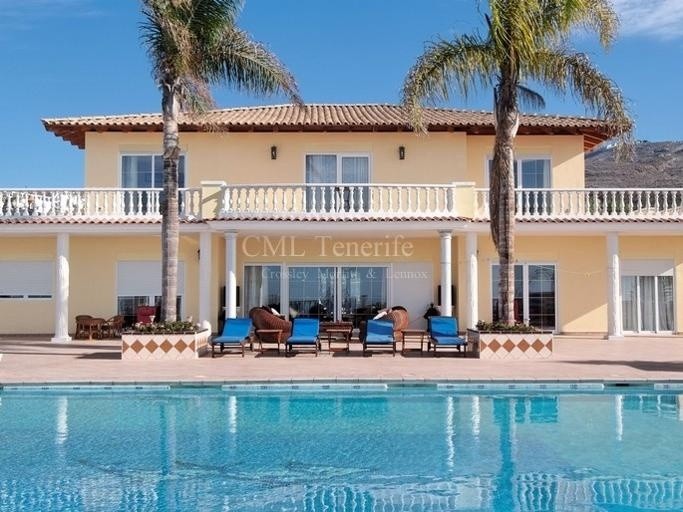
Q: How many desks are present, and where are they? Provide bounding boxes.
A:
[319,321,354,342]
[401,329,426,356]
[327,329,352,355]
[257,329,283,354]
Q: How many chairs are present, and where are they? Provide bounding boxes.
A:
[286,317,321,357]
[75,315,125,340]
[427,316,468,358]
[362,320,397,357]
[212,318,254,357]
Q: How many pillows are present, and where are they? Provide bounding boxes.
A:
[262,305,287,320]
[372,307,393,320]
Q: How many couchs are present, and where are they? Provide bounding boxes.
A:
[250,307,293,343]
[359,306,409,343]
